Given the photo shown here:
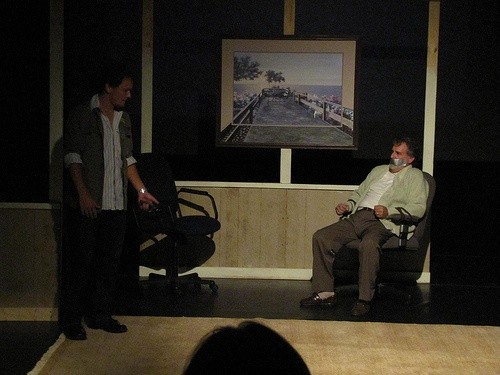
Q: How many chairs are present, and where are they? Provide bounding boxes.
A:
[311,172,436,303]
[123,153,221,304]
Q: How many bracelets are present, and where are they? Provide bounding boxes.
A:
[137,186,148,194]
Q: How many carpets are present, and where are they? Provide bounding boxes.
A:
[25,315,500,375]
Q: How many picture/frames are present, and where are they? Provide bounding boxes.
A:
[216,35,358,149]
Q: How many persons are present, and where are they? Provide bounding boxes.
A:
[62,70,162,341]
[181,321,311,375]
[299,135,430,317]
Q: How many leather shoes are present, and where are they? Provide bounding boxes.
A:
[88,316,128,333]
[300,293,338,306]
[351,302,376,316]
[59,323,86,340]
